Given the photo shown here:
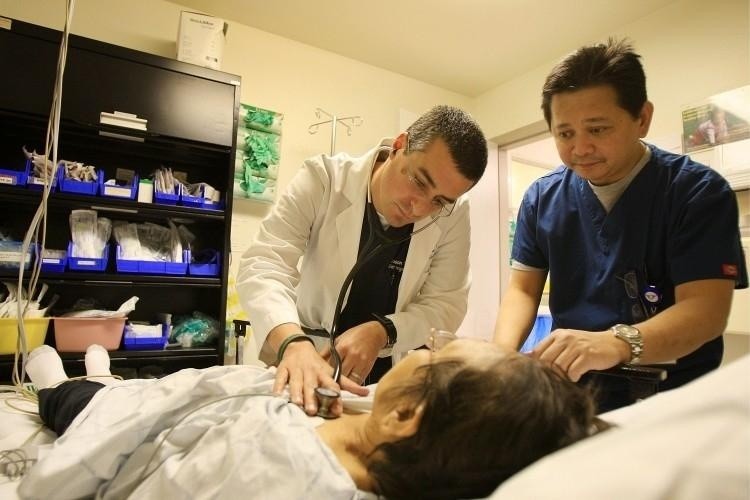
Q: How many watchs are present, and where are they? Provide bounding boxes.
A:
[608,323,644,366]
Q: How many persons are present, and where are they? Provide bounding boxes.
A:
[21,338,610,500]
[492,38,749,413]
[236,104,489,416]
[688,109,728,146]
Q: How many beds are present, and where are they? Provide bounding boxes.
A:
[0,365,750,499]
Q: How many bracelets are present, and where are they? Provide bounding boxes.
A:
[372,311,397,349]
[278,334,314,360]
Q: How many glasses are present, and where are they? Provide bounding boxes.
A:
[408,134,456,217]
[615,271,649,323]
[426,328,457,379]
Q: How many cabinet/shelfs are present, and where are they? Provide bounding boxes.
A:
[0,17,242,388]
